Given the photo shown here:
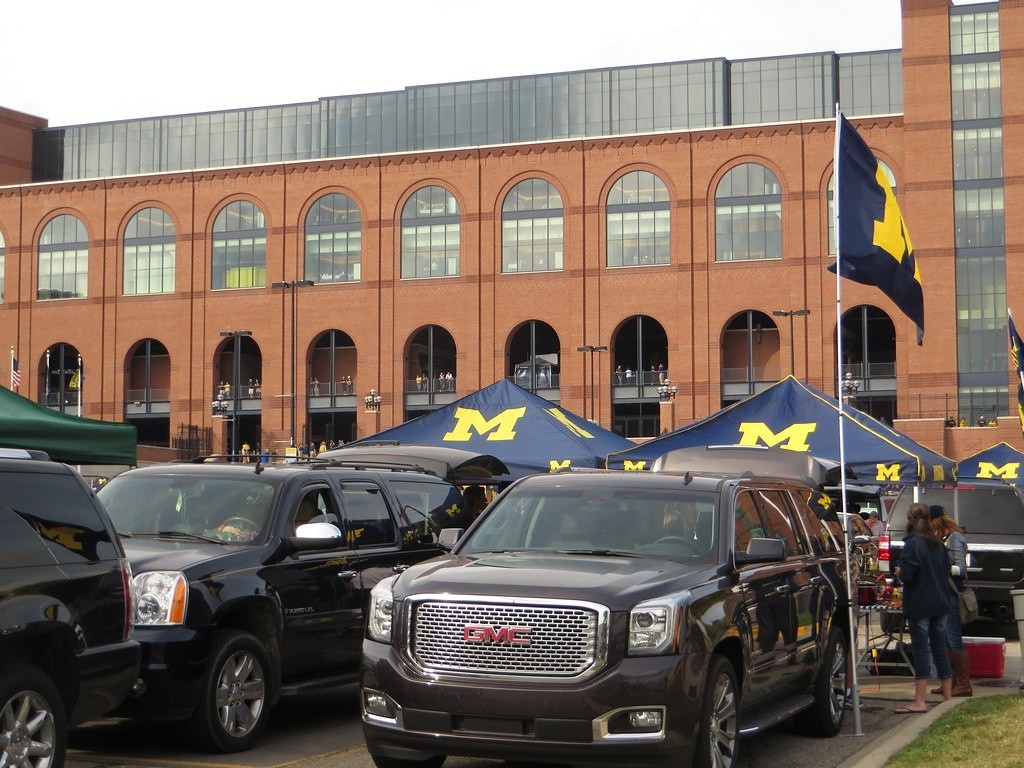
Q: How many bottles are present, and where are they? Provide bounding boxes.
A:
[883,582,901,603]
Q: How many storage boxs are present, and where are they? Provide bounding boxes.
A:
[961,637,1005,676]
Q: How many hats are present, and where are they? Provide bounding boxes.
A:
[869,511,878,518]
[928,505,946,520]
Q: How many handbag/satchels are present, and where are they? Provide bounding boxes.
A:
[959,587,979,626]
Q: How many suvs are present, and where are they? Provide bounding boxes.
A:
[359,440,858,768]
[0,447,144,768]
[875,478,1024,641]
[97,439,513,756]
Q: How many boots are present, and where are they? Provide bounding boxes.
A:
[951,650,972,696]
[931,648,957,694]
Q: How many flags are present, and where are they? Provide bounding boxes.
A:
[13,358,21,386]
[828,113,923,344]
[45,365,51,395]
[69,368,79,389]
[1007,314,1024,436]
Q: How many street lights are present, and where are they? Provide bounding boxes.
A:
[583,345,608,420]
[271,280,314,447]
[220,330,253,464]
[772,309,810,376]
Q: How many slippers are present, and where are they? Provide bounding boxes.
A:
[894,706,927,713]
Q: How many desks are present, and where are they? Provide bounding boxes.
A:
[857,607,915,686]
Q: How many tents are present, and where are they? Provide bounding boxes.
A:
[0,385,138,468]
[607,375,959,529]
[958,441,1024,493]
[315,379,637,480]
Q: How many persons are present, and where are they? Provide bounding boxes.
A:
[947,415,998,427]
[217,378,261,398]
[309,375,353,396]
[894,503,973,714]
[415,371,453,391]
[464,485,488,518]
[616,363,665,384]
[851,504,884,536]
[92,475,109,491]
[239,439,345,463]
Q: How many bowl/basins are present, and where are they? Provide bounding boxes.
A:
[858,586,878,604]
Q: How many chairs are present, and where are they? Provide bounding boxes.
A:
[193,483,320,535]
[556,504,713,549]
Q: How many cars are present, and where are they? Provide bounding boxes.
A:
[836,511,881,578]
